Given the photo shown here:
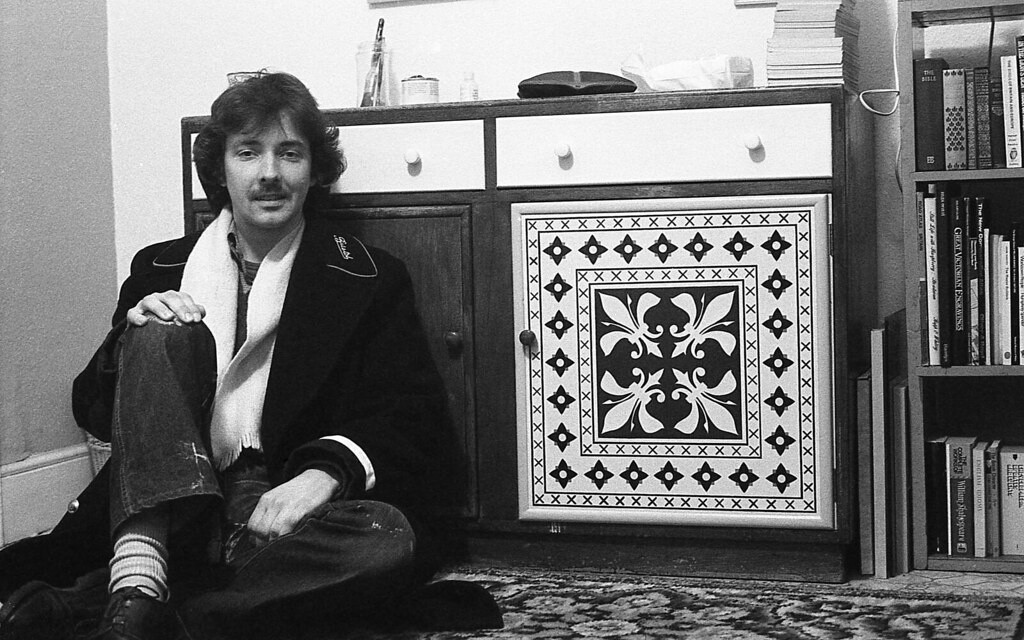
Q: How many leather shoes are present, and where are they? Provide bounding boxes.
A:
[88,585,175,640]
[0,569,112,640]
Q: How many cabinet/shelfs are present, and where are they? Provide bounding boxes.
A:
[895,0,1024,577]
[175,82,882,588]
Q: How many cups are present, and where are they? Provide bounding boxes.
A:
[227,72,269,87]
[401,80,438,105]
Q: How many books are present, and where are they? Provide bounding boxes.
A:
[766,0,860,88]
[916,184,1024,366]
[857,308,908,577]
[913,36,1024,171]
[929,436,1024,557]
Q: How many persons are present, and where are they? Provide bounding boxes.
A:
[0,69,502,640]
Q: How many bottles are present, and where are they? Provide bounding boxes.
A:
[355,38,394,108]
[462,72,481,102]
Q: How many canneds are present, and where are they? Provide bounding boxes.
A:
[401,77,439,104]
